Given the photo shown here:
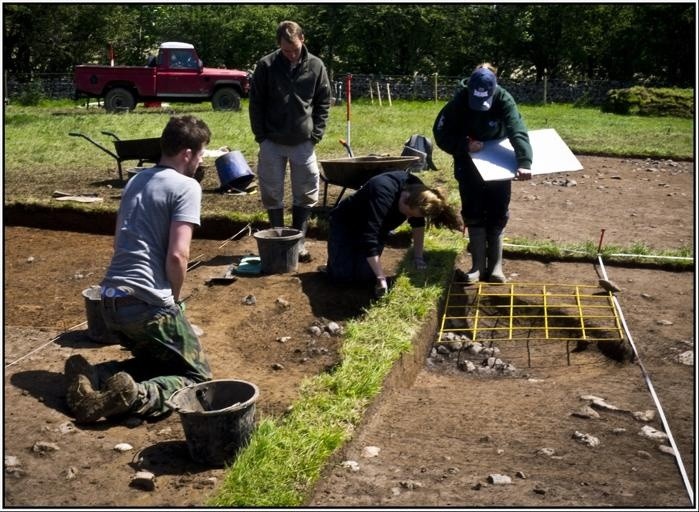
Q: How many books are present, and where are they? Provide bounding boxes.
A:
[467,128,584,182]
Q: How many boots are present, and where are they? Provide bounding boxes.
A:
[462,226,486,282]
[291,206,311,263]
[485,225,505,282]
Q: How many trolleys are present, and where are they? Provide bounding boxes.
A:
[313,140,428,190]
[64,128,163,185]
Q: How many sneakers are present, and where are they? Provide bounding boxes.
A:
[66,355,99,415]
[78,372,145,423]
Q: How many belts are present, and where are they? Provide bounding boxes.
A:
[100,295,145,306]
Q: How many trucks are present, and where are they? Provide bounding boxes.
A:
[70,38,250,113]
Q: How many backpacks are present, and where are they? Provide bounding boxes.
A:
[405,134,440,172]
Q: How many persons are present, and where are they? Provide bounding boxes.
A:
[433,67,532,283]
[248,20,331,265]
[63,114,214,429]
[317,170,464,300]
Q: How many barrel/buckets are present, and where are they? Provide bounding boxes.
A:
[215,149,256,194]
[79,281,107,341]
[166,378,260,469]
[254,227,306,276]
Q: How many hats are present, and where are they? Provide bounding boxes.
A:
[467,69,496,111]
